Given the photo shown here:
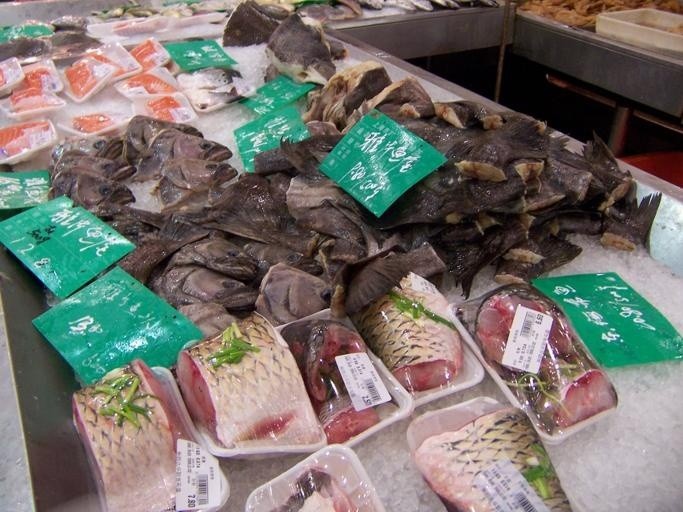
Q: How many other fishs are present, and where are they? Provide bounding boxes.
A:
[47,105,663,329]
